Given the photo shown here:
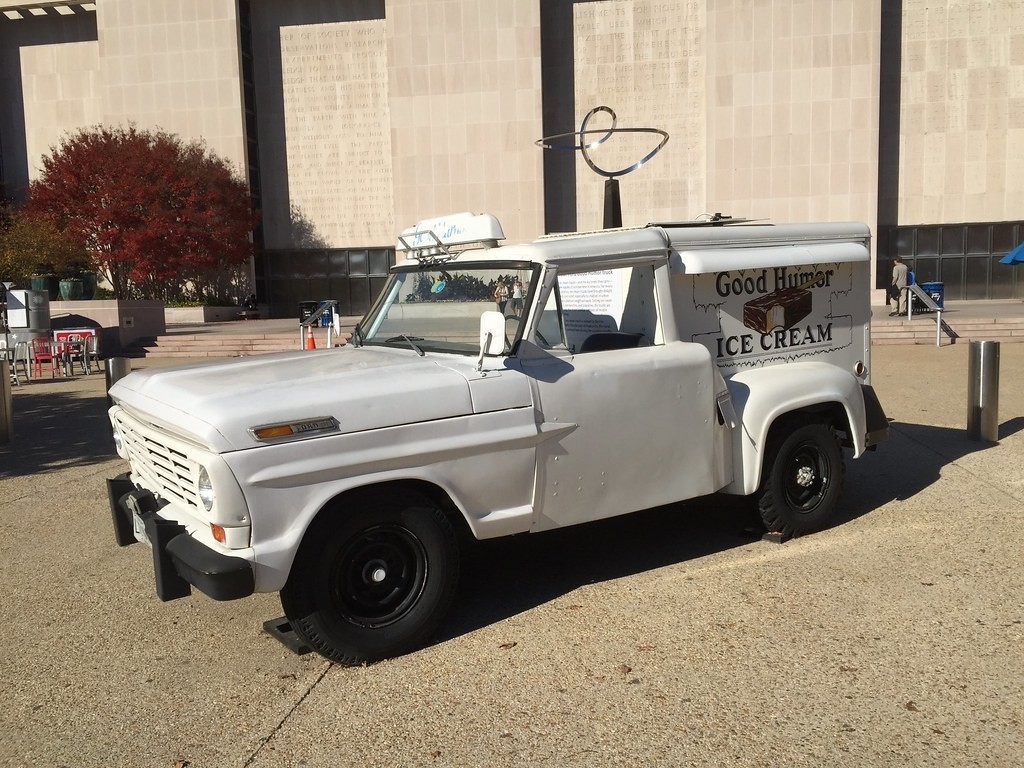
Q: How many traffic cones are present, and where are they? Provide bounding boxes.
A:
[306,322,317,350]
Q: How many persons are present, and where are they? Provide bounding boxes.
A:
[889,255,908,317]
[510,275,523,318]
[905,263,919,315]
[493,281,510,316]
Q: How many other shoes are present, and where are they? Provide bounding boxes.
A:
[889,312,898,316]
[898,312,907,316]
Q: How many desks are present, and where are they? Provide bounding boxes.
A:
[0,348,17,383]
[38,340,88,379]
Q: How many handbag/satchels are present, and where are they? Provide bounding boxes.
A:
[496,292,501,304]
[889,284,900,299]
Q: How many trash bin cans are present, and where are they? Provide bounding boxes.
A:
[920,282,944,311]
[320,299,339,326]
[299,301,318,327]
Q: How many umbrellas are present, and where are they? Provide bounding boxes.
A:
[998,240,1024,266]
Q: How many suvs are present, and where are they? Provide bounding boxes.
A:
[92,215,886,669]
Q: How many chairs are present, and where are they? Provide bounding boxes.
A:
[79,335,101,375]
[61,334,84,376]
[31,337,61,380]
[9,340,32,386]
